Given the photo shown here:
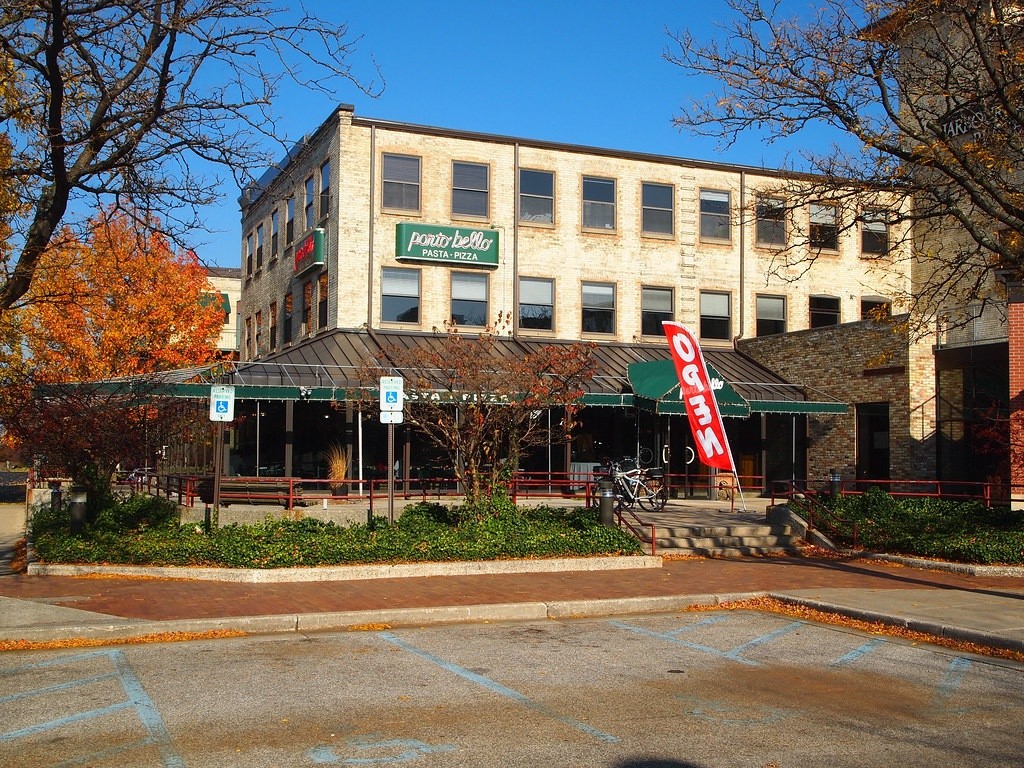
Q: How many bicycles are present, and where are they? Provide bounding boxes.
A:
[592,456,669,513]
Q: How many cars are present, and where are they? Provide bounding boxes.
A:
[124,466,154,484]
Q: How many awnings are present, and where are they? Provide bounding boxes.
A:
[34,359,848,415]
